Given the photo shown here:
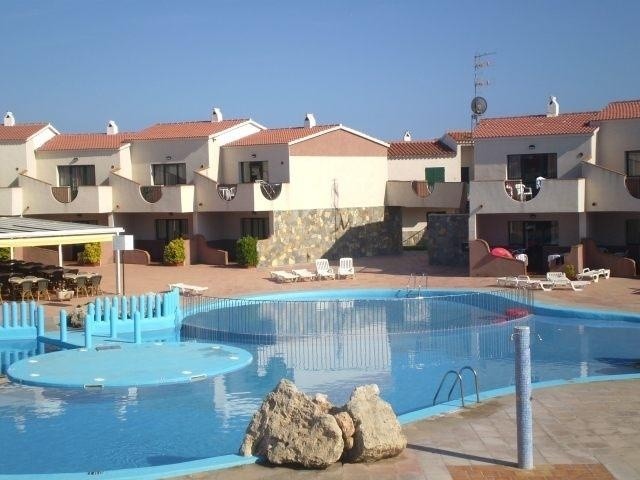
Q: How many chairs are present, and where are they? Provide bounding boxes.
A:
[169,282,208,297]
[0,257,104,303]
[270,257,355,285]
[496,248,611,292]
[506,176,547,202]
